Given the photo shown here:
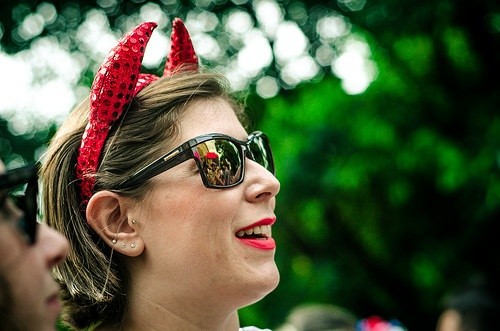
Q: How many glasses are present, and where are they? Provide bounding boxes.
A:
[0,163,38,246]
[112,131,274,189]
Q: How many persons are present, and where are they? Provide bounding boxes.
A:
[279,301,358,331]
[0,158,72,330]
[32,16,282,331]
